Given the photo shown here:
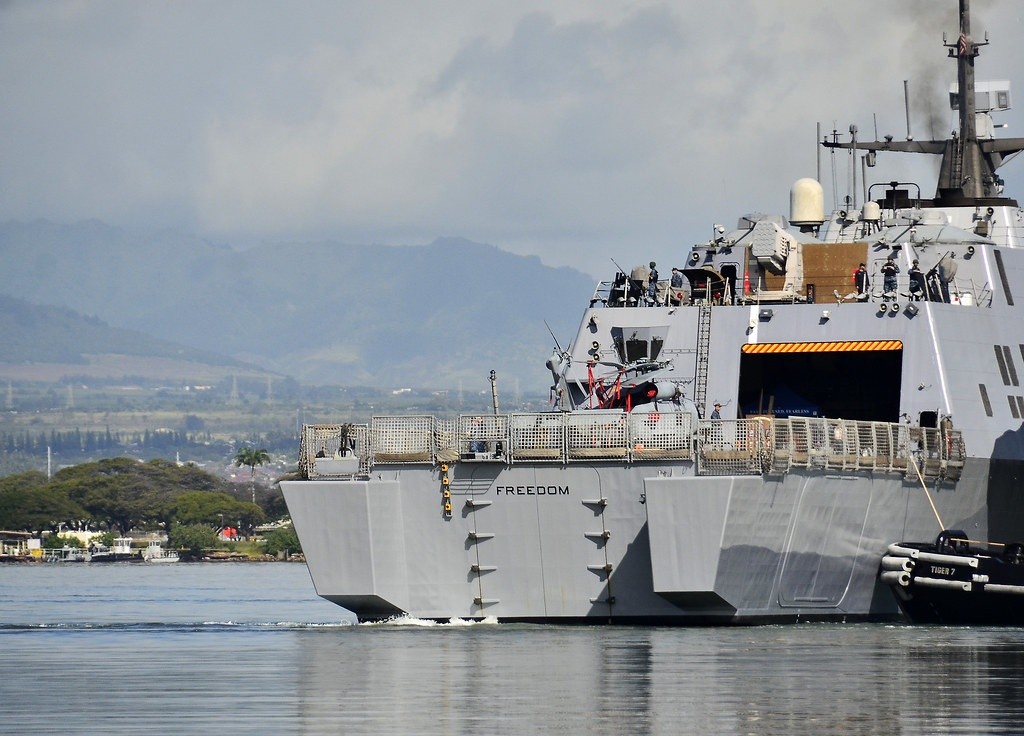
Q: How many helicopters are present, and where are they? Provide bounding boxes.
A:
[537,319,704,445]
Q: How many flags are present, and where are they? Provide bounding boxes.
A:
[614,377,621,400]
[959,23,967,56]
[588,365,594,396]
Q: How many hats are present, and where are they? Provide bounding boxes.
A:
[887,256,893,260]
[714,403,722,407]
[859,262,866,265]
[649,261,656,266]
[913,259,919,263]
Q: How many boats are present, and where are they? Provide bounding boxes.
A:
[872,533,1021,626]
[276,1,1024,630]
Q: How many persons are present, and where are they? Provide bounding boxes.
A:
[855,262,870,302]
[711,403,724,452]
[907,259,921,301]
[595,380,612,409]
[833,417,848,455]
[470,416,486,452]
[881,256,901,301]
[647,262,661,307]
